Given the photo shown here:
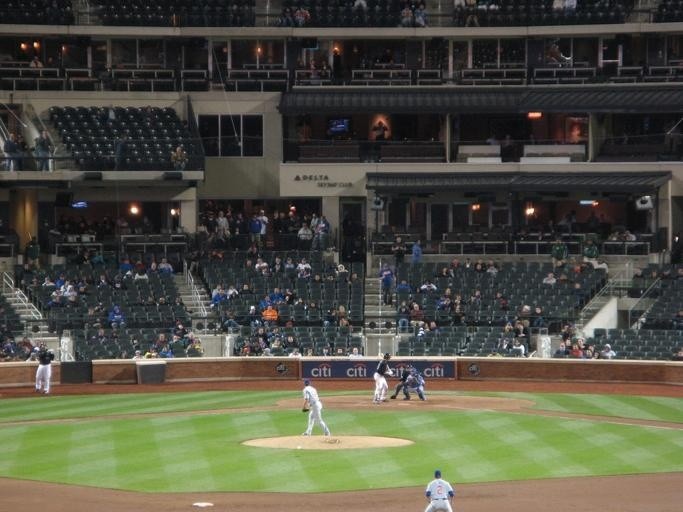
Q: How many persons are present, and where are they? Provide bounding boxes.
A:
[2,205,683,402]
[300,378,330,437]
[423,469,455,512]
[0,1,683,172]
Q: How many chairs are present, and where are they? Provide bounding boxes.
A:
[0,0,683,362]
